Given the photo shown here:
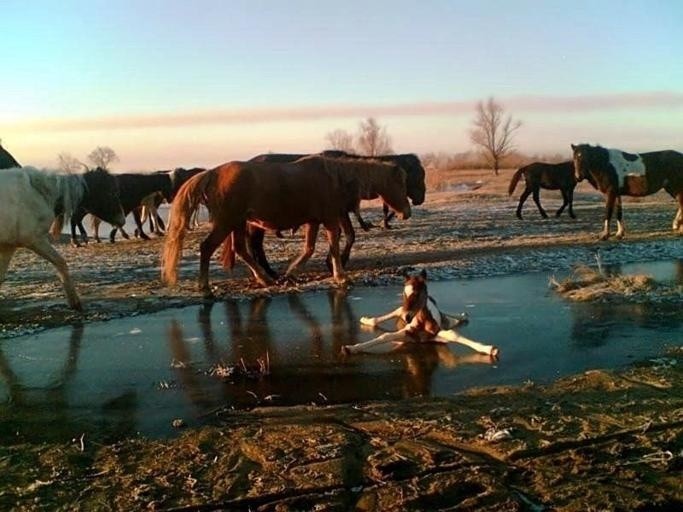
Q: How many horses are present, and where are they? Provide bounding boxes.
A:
[342,268,500,356]
[0,146,426,313]
[507,161,577,220]
[571,143,683,240]
